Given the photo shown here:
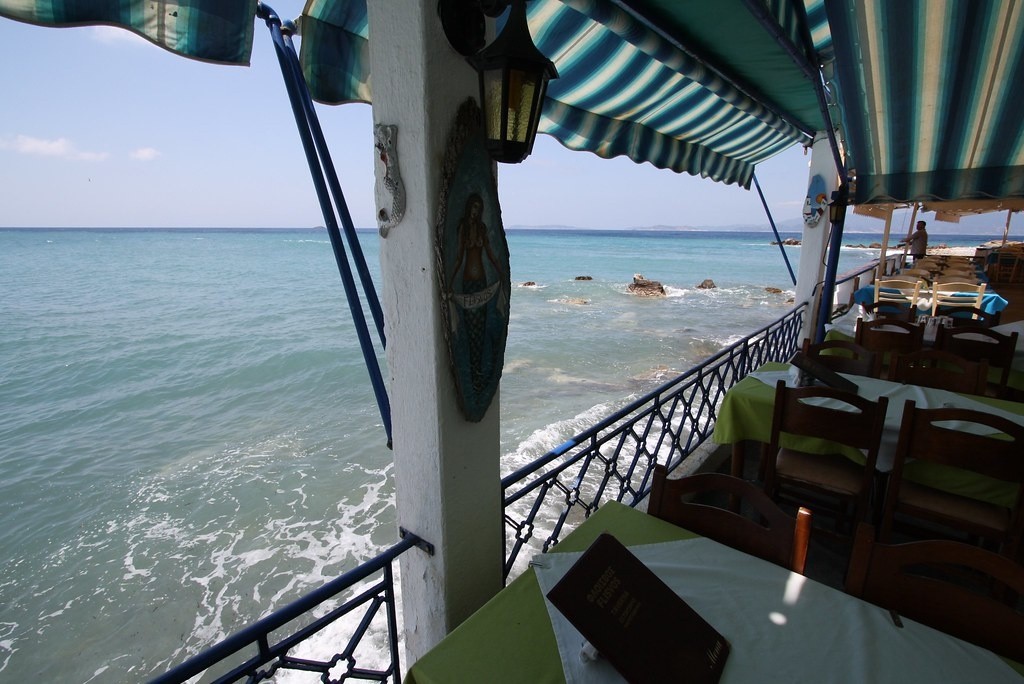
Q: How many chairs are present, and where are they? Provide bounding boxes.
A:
[994,248,1021,283]
[649,256,1024,664]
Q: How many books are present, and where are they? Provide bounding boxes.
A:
[790,352,859,395]
[546,530,731,684]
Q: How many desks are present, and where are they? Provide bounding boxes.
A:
[716,362,1024,550]
[399,498,1024,684]
[854,284,1009,320]
[819,302,1024,395]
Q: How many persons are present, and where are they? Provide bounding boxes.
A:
[900,221,927,262]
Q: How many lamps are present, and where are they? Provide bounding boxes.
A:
[827,196,849,225]
[437,1,561,163]
